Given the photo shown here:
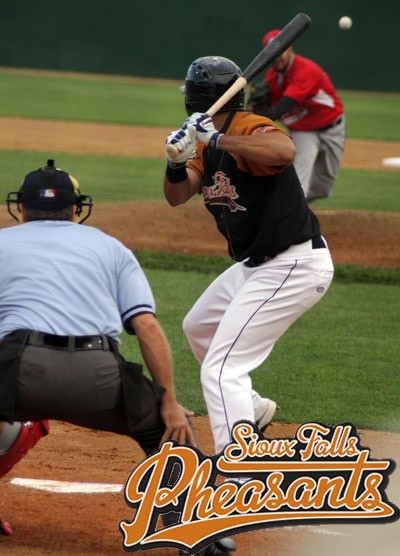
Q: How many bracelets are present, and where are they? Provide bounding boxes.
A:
[166,166,188,184]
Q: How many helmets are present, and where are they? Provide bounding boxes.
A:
[184,55,246,115]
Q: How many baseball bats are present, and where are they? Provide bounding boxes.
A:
[165,12,311,156]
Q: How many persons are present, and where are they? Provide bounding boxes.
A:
[1,158,239,555]
[245,28,348,201]
[163,53,336,487]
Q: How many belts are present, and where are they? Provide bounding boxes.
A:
[312,115,343,131]
[5,325,119,351]
[249,249,276,265]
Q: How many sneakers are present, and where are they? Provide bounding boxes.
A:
[179,540,236,556]
[255,397,277,435]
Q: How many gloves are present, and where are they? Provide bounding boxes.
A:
[166,126,198,165]
[182,111,221,151]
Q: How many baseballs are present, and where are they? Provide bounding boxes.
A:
[338,16,351,28]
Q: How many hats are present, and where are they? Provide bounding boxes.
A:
[19,165,81,210]
[261,31,294,55]
